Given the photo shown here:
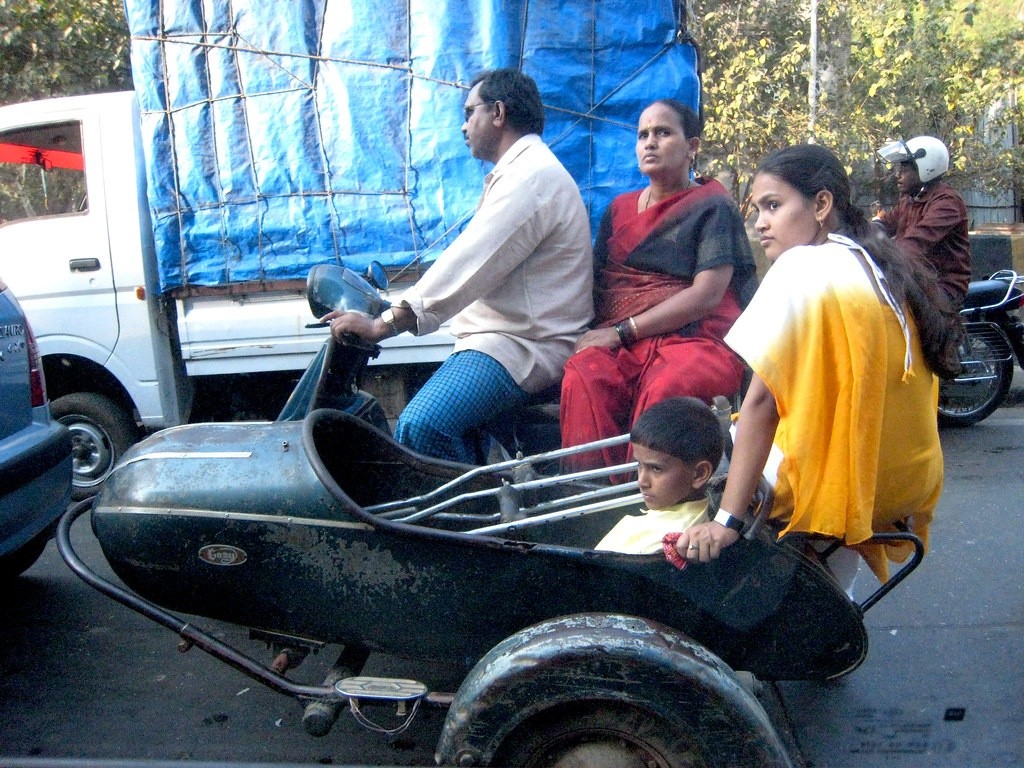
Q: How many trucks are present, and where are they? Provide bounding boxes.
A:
[0,1,703,502]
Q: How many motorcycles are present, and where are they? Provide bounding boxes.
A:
[57,259,926,768]
[936,269,1024,428]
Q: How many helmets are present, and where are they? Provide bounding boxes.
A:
[899,134,951,185]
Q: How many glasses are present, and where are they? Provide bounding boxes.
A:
[895,165,915,173]
[464,100,496,123]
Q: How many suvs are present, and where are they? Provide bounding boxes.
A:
[0,280,74,580]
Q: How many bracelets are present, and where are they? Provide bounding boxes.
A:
[615,320,636,348]
[629,316,639,339]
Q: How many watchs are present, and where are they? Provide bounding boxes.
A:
[708,508,745,530]
[380,308,399,336]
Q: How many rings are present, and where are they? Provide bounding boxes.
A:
[688,545,699,550]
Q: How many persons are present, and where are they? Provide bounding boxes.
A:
[319,69,595,526]
[593,395,725,555]
[867,135,971,334]
[556,103,759,488]
[676,141,945,600]
